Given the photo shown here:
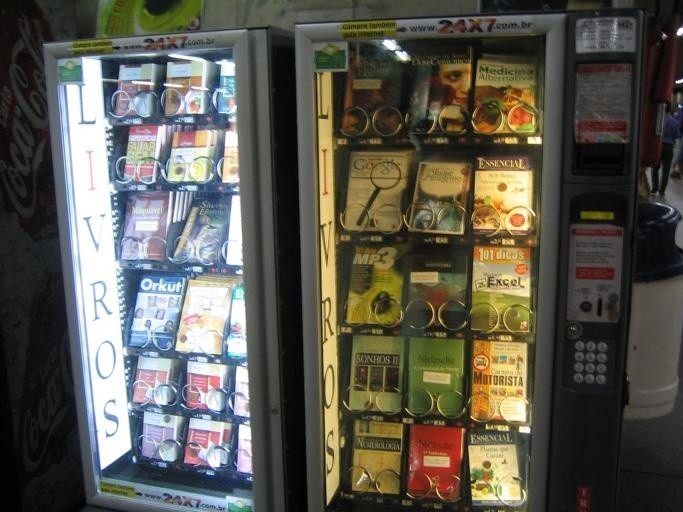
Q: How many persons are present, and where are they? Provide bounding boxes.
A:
[134,308,143,318]
[170,297,178,306]
[156,309,165,319]
[652,103,678,200]
[438,62,471,106]
[164,321,173,331]
[148,296,156,305]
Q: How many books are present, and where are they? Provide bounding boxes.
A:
[218,58,236,115]
[116,63,159,118]
[470,339,528,423]
[168,128,218,184]
[165,61,204,116]
[184,417,224,469]
[406,255,467,333]
[408,45,472,135]
[222,131,239,184]
[407,419,465,499]
[221,422,232,465]
[120,190,228,262]
[407,162,471,235]
[474,60,535,135]
[132,354,180,405]
[466,432,522,507]
[473,155,531,237]
[342,50,403,135]
[141,411,179,459]
[186,361,226,413]
[234,366,252,418]
[175,279,231,356]
[236,424,253,474]
[350,419,403,495]
[407,336,464,417]
[471,245,531,333]
[348,334,404,413]
[344,243,407,324]
[344,151,405,232]
[128,274,184,349]
[123,124,184,184]
